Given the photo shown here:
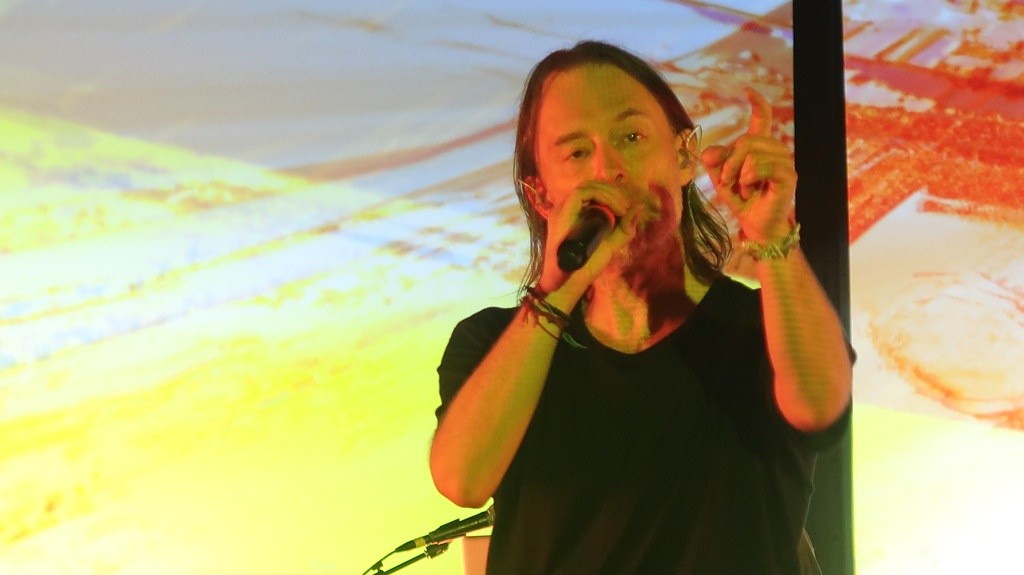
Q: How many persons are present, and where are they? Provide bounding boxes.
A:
[429,42,855,575]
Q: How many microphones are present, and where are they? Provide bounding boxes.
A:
[557,197,620,272]
[394,504,495,552]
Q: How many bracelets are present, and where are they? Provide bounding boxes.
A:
[743,224,797,260]
[522,289,571,339]
